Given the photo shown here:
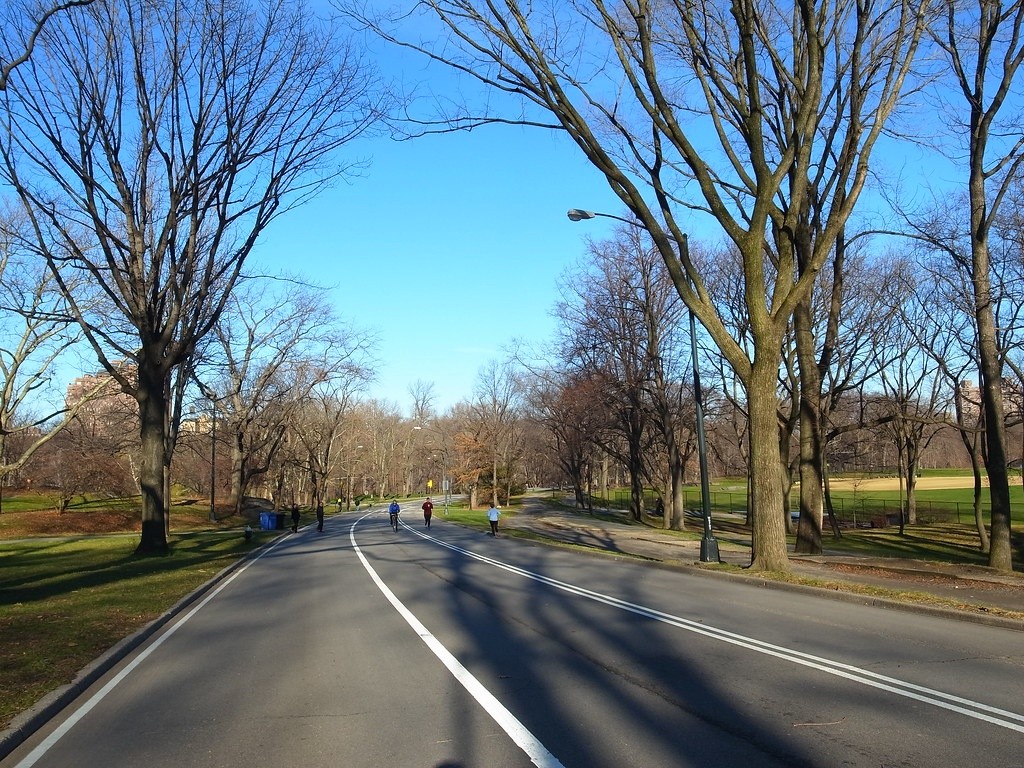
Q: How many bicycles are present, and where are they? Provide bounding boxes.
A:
[391,513,400,532]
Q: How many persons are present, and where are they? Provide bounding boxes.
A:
[355,499,360,510]
[487,504,501,536]
[290,504,300,533]
[336,501,342,512]
[422,497,433,527]
[317,502,323,532]
[389,500,400,526]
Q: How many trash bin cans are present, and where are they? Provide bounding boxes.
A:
[277,511,292,530]
[260,513,277,531]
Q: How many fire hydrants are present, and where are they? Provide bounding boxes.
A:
[244,525,253,544]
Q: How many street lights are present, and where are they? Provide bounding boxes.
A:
[567,208,727,566]
[414,426,450,516]
[208,387,258,520]
